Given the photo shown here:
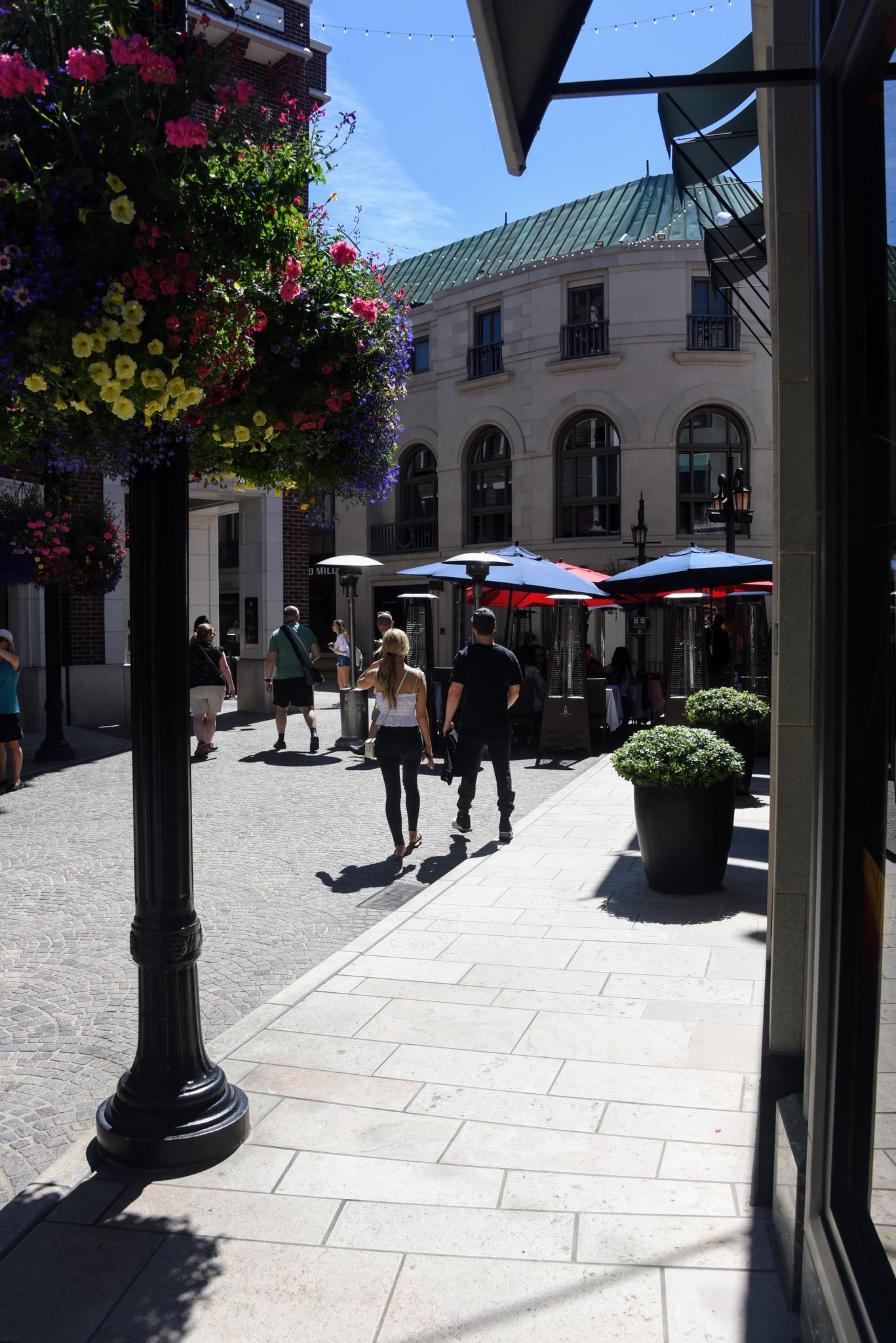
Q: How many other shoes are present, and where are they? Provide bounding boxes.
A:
[5,781,24,792]
[273,739,287,748]
[309,733,319,751]
[0,778,8,793]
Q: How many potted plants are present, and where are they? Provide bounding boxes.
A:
[613,718,748,897]
[683,680,771,794]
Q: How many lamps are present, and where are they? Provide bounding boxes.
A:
[478,269,489,276]
[595,240,605,247]
[657,232,666,240]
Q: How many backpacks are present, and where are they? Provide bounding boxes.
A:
[339,633,363,666]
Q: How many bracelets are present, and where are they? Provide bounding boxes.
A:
[265,677,271,682]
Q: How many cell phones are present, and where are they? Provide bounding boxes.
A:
[423,751,430,761]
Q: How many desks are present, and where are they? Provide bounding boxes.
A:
[608,685,620,745]
[643,678,661,726]
[625,682,645,727]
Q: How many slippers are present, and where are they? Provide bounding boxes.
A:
[393,846,408,859]
[410,833,422,846]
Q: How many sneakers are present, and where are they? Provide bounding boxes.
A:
[205,742,219,752]
[499,824,514,839]
[195,741,209,756]
[452,816,471,830]
[332,701,341,707]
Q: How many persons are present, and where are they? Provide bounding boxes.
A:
[188,623,235,759]
[0,629,25,792]
[266,606,322,752]
[514,644,639,731]
[443,607,524,840]
[328,619,350,707]
[704,614,732,678]
[194,615,210,632]
[349,611,394,756]
[228,618,240,655]
[356,628,433,860]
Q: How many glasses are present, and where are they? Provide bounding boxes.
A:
[209,632,216,637]
[0,637,8,642]
[204,620,209,623]
[377,611,390,617]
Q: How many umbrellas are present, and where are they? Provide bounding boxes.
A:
[465,556,659,607]
[597,540,773,691]
[654,581,773,626]
[395,540,628,647]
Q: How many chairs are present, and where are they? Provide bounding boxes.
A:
[438,673,466,749]
[509,677,541,750]
[587,678,606,751]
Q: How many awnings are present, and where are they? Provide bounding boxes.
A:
[702,204,772,357]
[657,32,763,256]
[466,0,896,177]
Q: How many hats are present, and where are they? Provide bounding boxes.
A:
[471,607,496,629]
[0,629,15,654]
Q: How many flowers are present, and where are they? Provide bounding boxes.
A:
[0,0,430,600]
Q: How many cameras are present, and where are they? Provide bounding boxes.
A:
[327,642,334,648]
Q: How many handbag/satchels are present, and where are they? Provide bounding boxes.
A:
[364,738,377,759]
[303,663,325,687]
